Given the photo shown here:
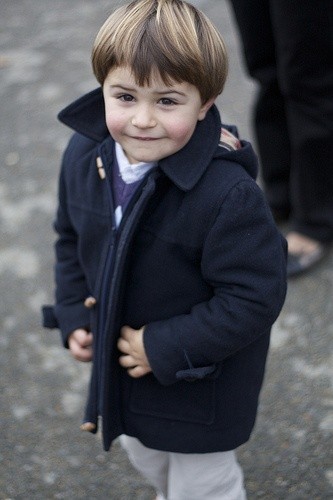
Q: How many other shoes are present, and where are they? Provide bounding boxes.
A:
[287,228,328,275]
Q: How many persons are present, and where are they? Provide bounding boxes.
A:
[43,1,290,499]
[228,2,333,280]
